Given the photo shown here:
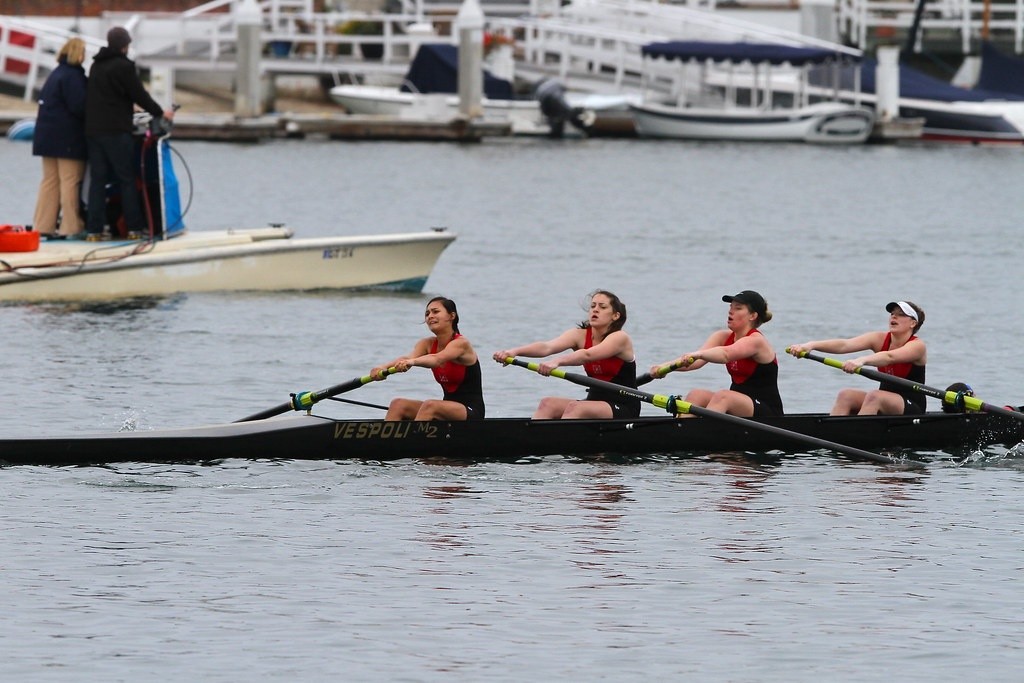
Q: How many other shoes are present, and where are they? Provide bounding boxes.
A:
[41,232,59,240]
[60,230,88,240]
[85,233,100,242]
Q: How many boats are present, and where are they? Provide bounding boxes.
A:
[0,404,1024,465]
[0,224,457,302]
[329,39,1024,144]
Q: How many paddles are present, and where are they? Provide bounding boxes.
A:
[232,365,407,423]
[505,356,897,465]
[785,346,1024,423]
[633,357,695,392]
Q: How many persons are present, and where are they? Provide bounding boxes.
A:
[369,297,486,422]
[650,290,784,419]
[493,289,642,419]
[33,38,92,236]
[789,301,927,416]
[83,26,174,242]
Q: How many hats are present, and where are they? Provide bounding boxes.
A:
[722,290,765,324]
[108,28,132,50]
[886,301,919,326]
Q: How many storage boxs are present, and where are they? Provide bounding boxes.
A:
[0,224,39,251]
